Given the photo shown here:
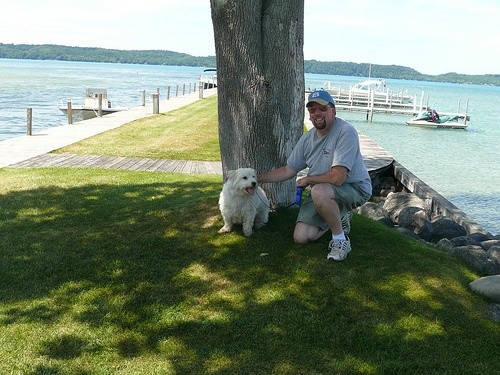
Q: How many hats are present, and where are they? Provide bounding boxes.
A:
[306,91,335,107]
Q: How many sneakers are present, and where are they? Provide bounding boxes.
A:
[341,211,353,234]
[326,235,352,261]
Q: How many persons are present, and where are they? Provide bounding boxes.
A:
[256,91,372,261]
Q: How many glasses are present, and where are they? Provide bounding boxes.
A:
[308,106,329,113]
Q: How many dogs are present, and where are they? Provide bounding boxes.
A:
[217,168,269,235]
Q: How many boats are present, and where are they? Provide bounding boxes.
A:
[350,80,414,104]
[404,110,469,129]
[198,67,217,89]
[58,86,130,120]
